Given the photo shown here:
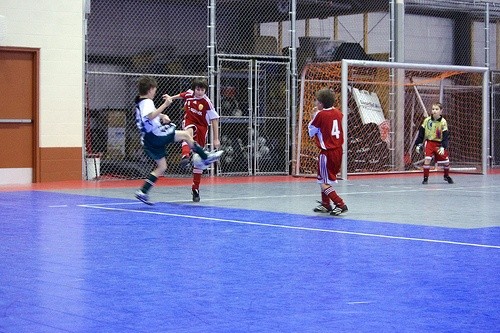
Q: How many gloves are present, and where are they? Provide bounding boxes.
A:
[436,147,445,156]
[415,145,421,154]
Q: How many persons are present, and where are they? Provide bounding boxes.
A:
[134,77,225,206]
[308,89,348,216]
[415,102,454,184]
[162,78,220,202]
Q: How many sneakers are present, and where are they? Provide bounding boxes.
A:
[422,176,429,186]
[443,174,455,184]
[180,156,190,170]
[135,190,155,206]
[203,151,223,165]
[191,188,200,202]
[313,204,333,213]
[330,206,348,216]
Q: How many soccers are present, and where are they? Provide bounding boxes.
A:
[194,151,210,170]
